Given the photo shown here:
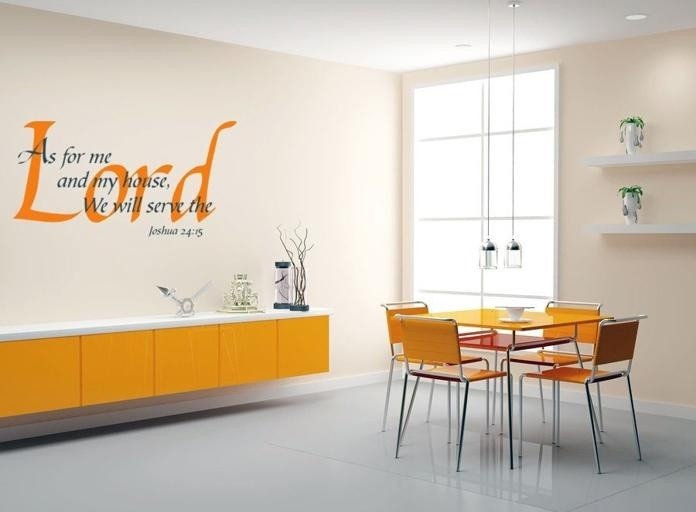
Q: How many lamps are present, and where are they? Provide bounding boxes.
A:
[502,0,523,269]
[480,1,499,269]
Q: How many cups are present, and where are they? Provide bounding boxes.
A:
[508,307,523,321]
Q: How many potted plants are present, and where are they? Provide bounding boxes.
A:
[618,116,646,154]
[618,184,644,226]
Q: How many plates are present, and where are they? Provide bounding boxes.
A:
[500,317,530,322]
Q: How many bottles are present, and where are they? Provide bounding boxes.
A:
[274,262,293,309]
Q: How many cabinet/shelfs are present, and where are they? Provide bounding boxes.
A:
[0,309,335,418]
[586,151,695,234]
